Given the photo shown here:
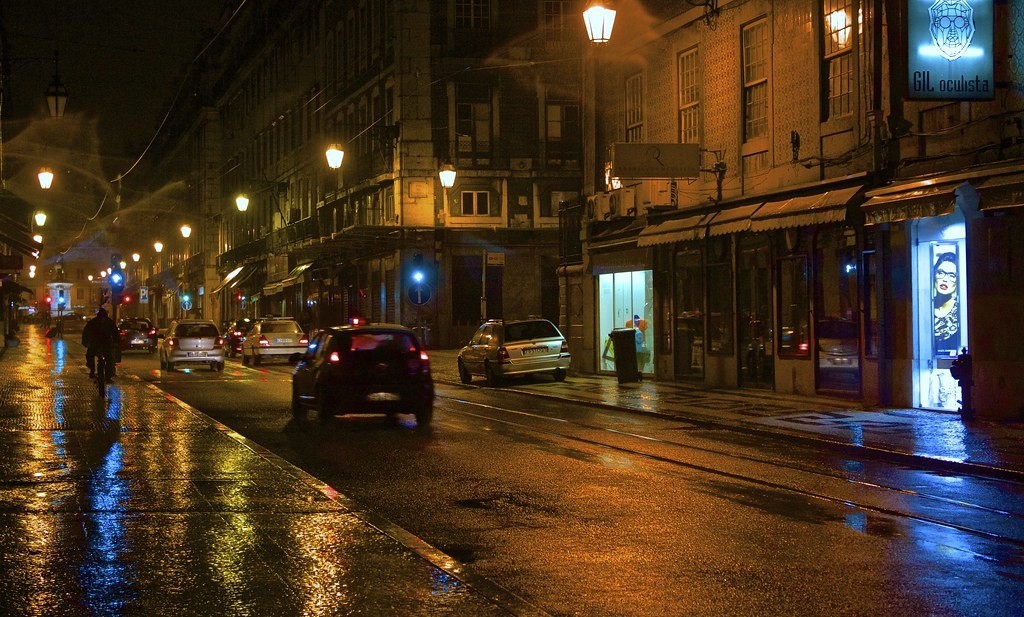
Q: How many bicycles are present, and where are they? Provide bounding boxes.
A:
[95,353,109,398]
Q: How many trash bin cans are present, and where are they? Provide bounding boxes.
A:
[609,327,642,384]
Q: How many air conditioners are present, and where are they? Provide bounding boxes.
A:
[609,188,635,220]
[587,194,610,222]
[642,178,678,209]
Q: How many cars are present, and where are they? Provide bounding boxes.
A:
[457,314,571,388]
[290,318,436,429]
[156,319,225,371]
[22,312,52,323]
[241,319,309,366]
[117,317,157,354]
[85,311,110,321]
[54,312,86,321]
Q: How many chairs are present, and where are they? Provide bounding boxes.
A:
[328,335,354,353]
[176,326,187,336]
[262,324,273,333]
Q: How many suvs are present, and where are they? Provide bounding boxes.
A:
[223,320,255,356]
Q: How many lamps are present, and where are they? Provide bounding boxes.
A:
[34,209,47,227]
[180,223,192,238]
[235,175,289,212]
[154,239,163,253]
[580,0,617,43]
[37,166,54,192]
[132,252,140,262]
[325,143,344,169]
[440,164,457,188]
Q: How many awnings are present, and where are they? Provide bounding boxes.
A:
[208,263,314,295]
[637,170,1024,246]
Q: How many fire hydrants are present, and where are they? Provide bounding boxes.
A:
[950,348,972,422]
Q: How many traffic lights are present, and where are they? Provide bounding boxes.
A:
[58,290,64,303]
[47,295,51,302]
[125,297,131,303]
[35,301,38,307]
[110,254,123,287]
[412,253,425,285]
[183,289,189,301]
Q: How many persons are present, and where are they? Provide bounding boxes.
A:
[931,252,960,352]
[82,309,120,384]
